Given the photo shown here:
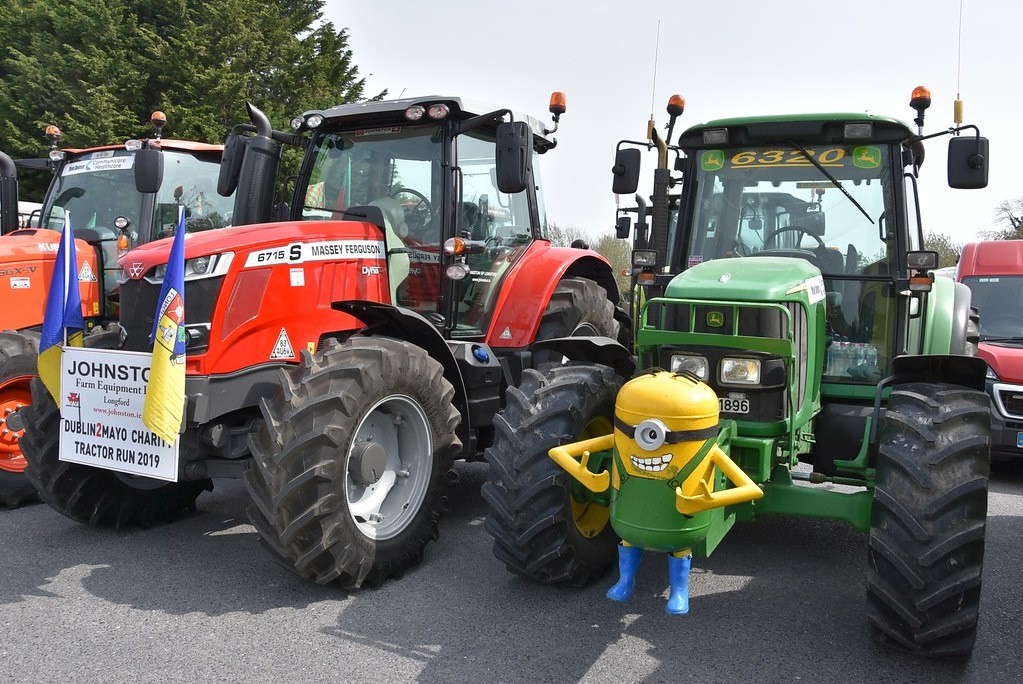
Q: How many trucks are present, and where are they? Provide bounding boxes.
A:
[948,240,1022,466]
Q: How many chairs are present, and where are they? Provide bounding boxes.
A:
[430,200,484,248]
[805,245,846,310]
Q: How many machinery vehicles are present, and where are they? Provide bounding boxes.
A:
[88,95,630,593]
[0,112,290,506]
[483,82,995,664]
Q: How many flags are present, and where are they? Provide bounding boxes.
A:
[304,182,324,211]
[38,215,83,410]
[331,160,351,220]
[142,207,186,446]
